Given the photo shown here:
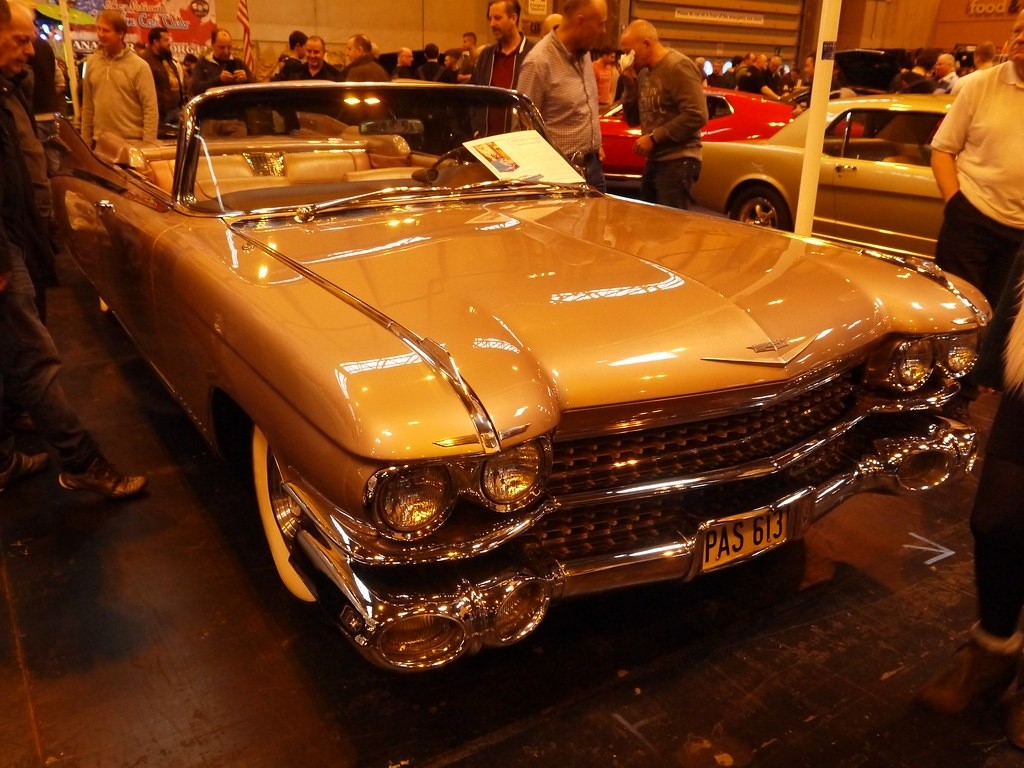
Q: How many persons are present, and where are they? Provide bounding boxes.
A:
[694,51,816,120]
[914,237,1024,751]
[620,19,708,211]
[133,25,258,139]
[468,0,537,136]
[540,13,563,37]
[511,0,608,192]
[268,31,495,146]
[79,9,160,166]
[927,6,1024,393]
[862,41,997,138]
[593,49,622,110]
[0,0,146,499]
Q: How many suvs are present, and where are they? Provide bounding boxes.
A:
[830,48,911,92]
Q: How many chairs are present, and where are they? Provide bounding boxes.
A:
[882,155,916,165]
[192,176,290,201]
[343,166,428,182]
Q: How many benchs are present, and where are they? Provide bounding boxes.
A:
[149,147,372,196]
[823,137,905,162]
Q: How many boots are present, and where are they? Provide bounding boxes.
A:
[922,620,1024,716]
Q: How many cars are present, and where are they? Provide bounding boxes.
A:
[595,84,800,191]
[44,76,995,670]
[687,92,957,262]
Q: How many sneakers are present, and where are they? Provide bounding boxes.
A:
[0,452,49,494]
[57,456,146,500]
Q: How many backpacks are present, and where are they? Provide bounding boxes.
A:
[722,66,747,89]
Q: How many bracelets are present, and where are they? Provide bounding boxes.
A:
[648,130,656,143]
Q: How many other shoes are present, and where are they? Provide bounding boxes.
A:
[944,398,972,426]
